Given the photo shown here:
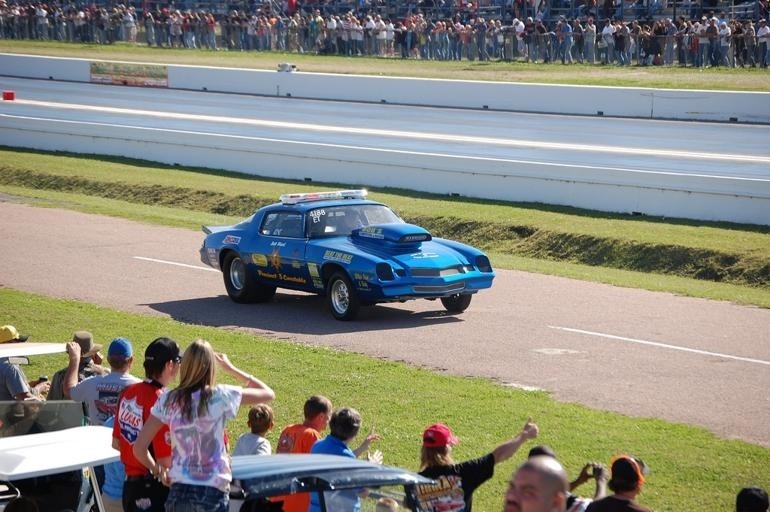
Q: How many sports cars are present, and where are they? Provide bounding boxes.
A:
[198,188,495,321]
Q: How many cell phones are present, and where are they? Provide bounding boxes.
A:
[39,376,48,383]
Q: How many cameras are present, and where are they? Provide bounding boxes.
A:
[593,466,601,477]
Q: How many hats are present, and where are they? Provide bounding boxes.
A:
[422,424,460,447]
[0,325,28,342]
[143,337,186,363]
[611,455,650,483]
[65,331,104,357]
[108,338,133,359]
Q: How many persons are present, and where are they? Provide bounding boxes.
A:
[340,209,361,231]
